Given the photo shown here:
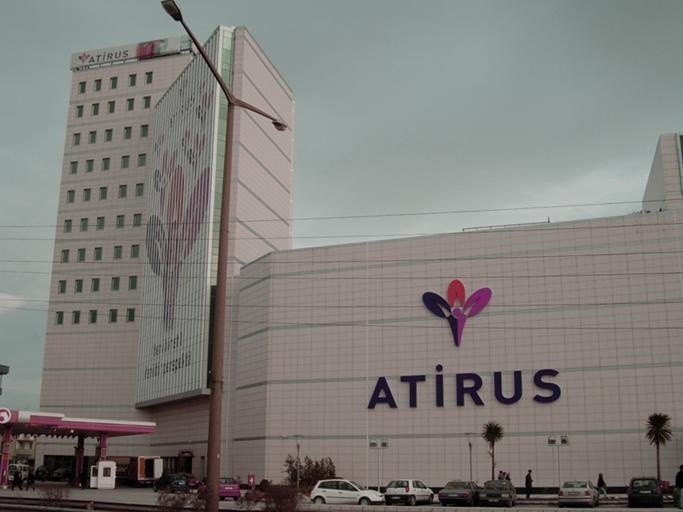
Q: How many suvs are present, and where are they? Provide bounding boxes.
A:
[626,477,662,507]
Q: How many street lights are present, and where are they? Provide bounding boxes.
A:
[161,0,289,509]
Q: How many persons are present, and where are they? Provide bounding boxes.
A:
[499,470,511,483]
[675,464,683,508]
[10,466,24,490]
[597,472,607,498]
[25,463,36,491]
[525,470,533,499]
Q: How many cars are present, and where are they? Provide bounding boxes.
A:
[558,479,599,507]
[153,472,242,503]
[437,481,478,506]
[383,479,433,506]
[479,480,516,508]
[7,462,72,484]
[308,477,384,506]
